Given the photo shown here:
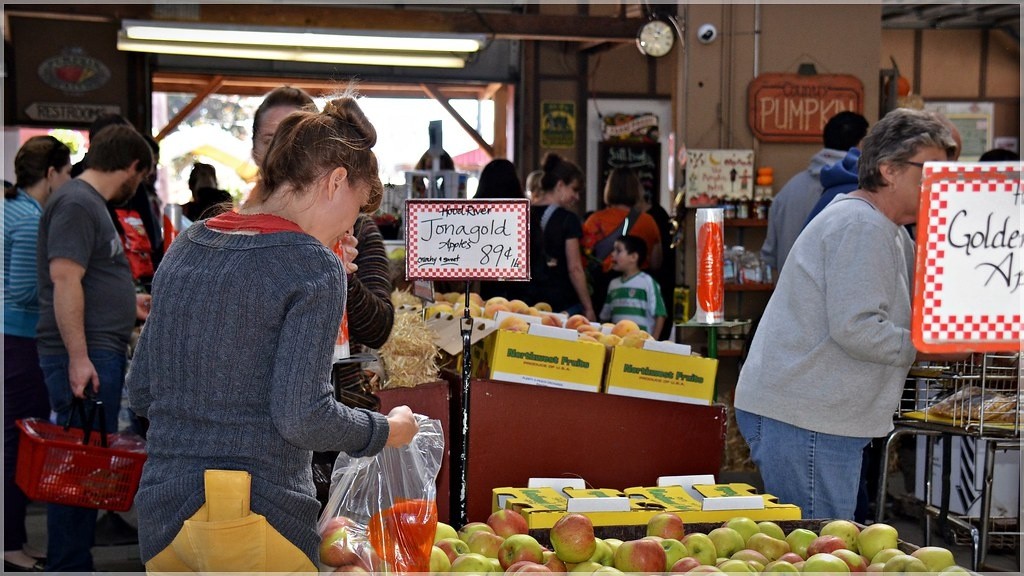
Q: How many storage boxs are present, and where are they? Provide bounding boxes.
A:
[604,344,719,406]
[482,328,606,394]
[490,473,803,531]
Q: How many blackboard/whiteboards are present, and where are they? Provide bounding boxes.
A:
[405,200,532,281]
[911,161,1024,353]
[686,149,756,208]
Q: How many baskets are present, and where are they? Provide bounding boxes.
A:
[17,387,148,512]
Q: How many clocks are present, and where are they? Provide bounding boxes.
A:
[639,20,674,57]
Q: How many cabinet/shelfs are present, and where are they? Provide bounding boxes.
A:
[719,217,772,361]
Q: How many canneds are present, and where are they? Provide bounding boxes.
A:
[720,195,764,219]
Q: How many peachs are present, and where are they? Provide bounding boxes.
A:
[423,292,657,349]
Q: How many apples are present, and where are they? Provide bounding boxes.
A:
[318,503,971,576]
[386,248,405,260]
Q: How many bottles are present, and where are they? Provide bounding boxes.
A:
[717,318,752,351]
[723,204,765,220]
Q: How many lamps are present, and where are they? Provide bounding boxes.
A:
[114,18,488,71]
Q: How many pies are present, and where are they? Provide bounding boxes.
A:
[929,385,1019,423]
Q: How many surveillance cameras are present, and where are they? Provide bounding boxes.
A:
[697,23,717,43]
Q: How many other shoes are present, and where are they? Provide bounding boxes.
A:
[4,550,47,572]
[870,496,893,507]
[864,508,890,526]
[91,514,138,546]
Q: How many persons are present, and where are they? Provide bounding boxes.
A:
[248,82,394,515]
[4,114,234,574]
[120,99,421,575]
[599,235,669,341]
[470,152,675,324]
[733,108,1017,534]
